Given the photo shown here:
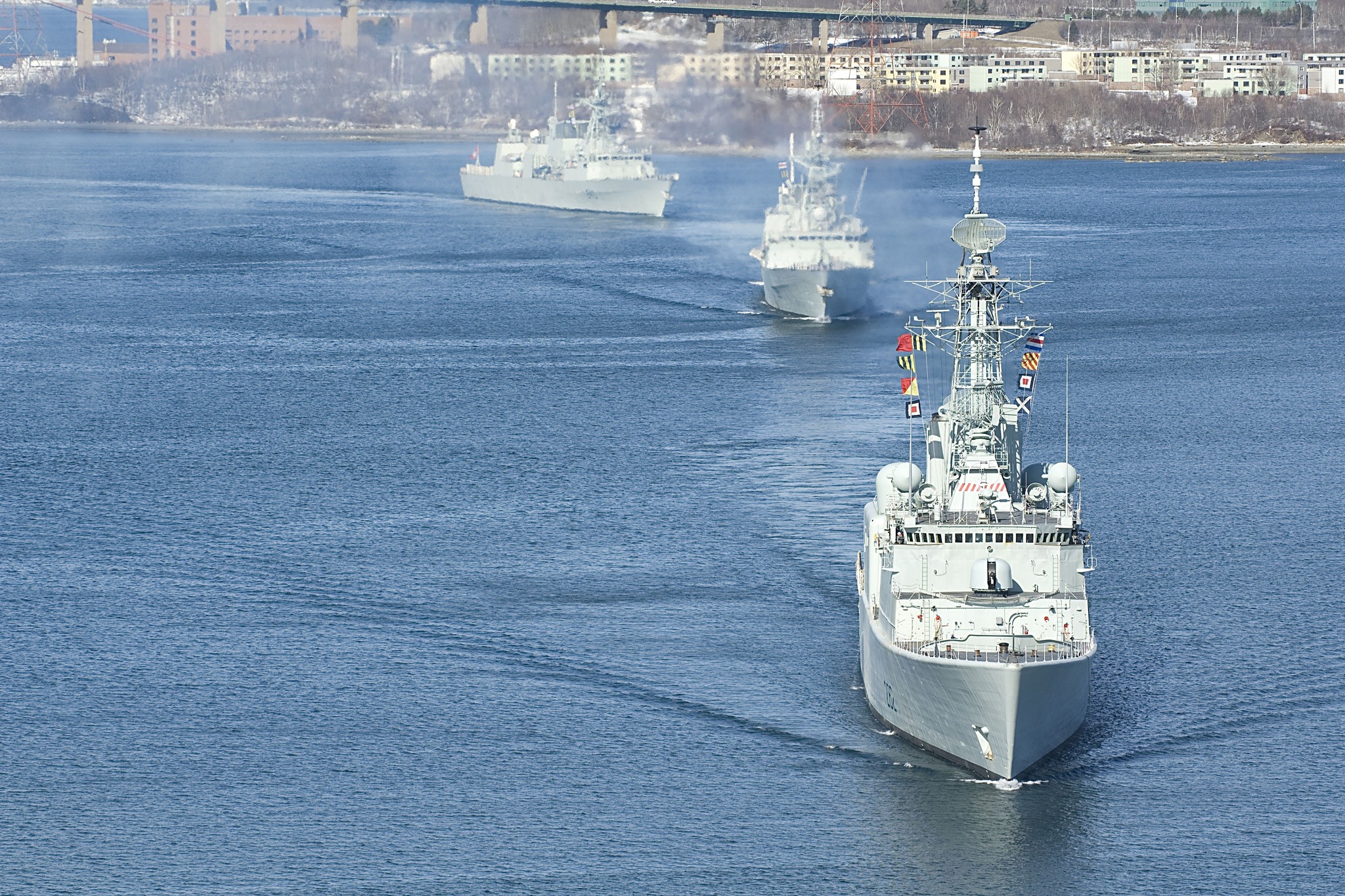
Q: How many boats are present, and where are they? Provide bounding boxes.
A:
[460,46,678,218]
[854,110,1098,779]
[750,80,875,321]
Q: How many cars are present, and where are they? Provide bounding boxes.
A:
[1008,15,1019,19]
[750,3,762,9]
[1080,16,1097,20]
[648,0,676,5]
[814,7,826,11]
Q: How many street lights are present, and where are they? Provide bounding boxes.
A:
[1197,24,1202,51]
[1296,3,1302,29]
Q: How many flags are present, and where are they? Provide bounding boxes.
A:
[896,333,913,353]
[909,333,927,352]
[905,399,922,419]
[900,376,919,396]
[1017,373,1036,392]
[895,353,916,373]
[1020,351,1041,371]
[1013,396,1031,414]
[1024,332,1045,351]
